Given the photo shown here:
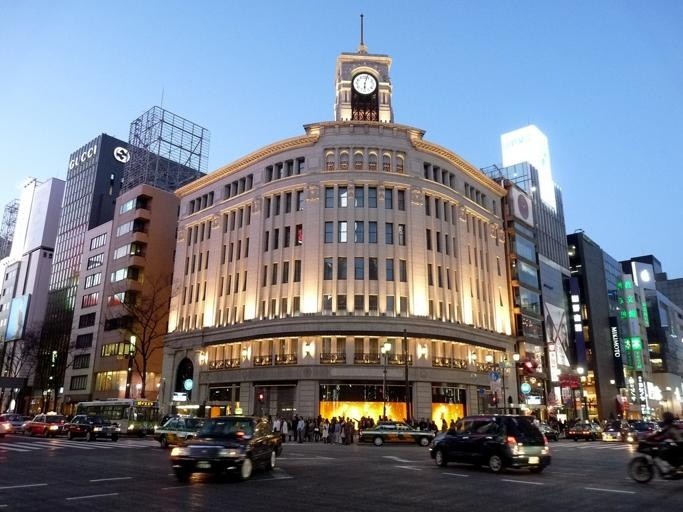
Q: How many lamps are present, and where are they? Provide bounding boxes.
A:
[421,342,427,359]
[242,346,247,360]
[472,351,477,362]
[200,352,204,365]
[305,342,310,355]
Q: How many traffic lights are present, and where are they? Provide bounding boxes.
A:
[257,390,265,402]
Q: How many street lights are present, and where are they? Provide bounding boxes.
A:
[608,374,635,419]
[124,336,138,399]
[554,365,586,420]
[44,349,57,412]
[483,351,521,414]
[377,340,392,420]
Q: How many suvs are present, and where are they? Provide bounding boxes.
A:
[428,413,551,476]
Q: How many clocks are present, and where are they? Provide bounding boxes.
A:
[352,71,378,95]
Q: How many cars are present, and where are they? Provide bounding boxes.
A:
[538,419,682,442]
[171,417,284,485]
[152,415,202,448]
[0,397,160,443]
[359,422,434,448]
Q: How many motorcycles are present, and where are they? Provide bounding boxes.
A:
[626,432,682,484]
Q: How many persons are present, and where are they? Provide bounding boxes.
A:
[272,413,393,448]
[403,416,463,435]
[530,408,683,469]
